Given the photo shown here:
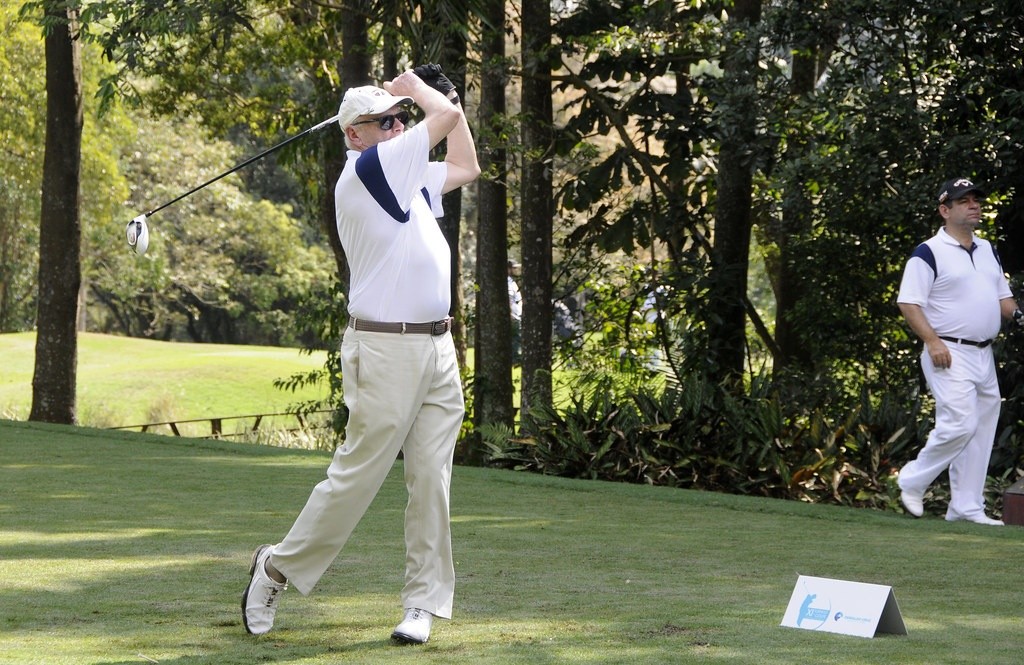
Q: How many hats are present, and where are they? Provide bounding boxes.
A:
[338,85,414,133]
[938,178,986,203]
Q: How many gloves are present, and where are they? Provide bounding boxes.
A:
[412,64,456,97]
[1013,309,1024,332]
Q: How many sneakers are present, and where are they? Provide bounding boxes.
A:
[391,608,432,643]
[241,544,289,634]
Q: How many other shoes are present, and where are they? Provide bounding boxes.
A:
[902,490,923,517]
[946,510,1005,526]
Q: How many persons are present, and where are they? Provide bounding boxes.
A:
[241,64,480,647]
[506,259,670,377]
[896,180,1024,526]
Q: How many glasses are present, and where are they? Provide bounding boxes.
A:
[352,111,410,130]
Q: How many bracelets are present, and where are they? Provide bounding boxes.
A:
[450,94,461,105]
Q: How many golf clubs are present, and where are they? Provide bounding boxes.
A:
[123,63,443,255]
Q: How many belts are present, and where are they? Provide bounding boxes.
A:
[938,336,993,347]
[349,316,454,336]
